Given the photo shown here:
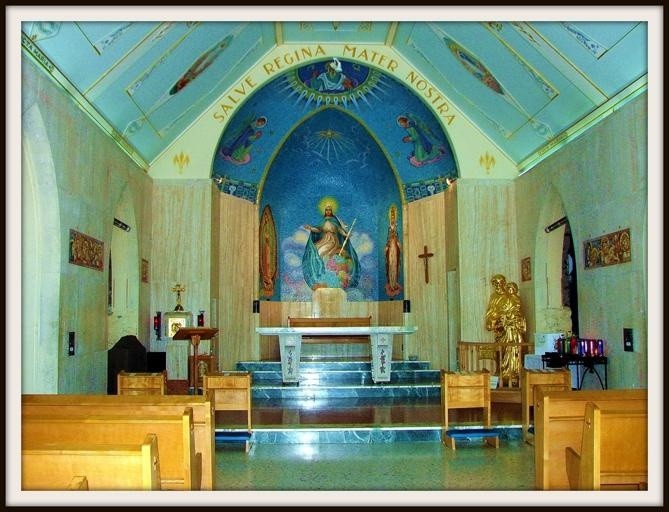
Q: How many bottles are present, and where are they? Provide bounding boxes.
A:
[555,330,604,357]
[197,310,205,327]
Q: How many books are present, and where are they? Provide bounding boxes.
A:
[556,336,603,357]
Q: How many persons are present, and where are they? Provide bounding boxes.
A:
[485,275,527,376]
[396,112,446,167]
[220,116,267,165]
[498,282,522,330]
[312,58,354,92]
[304,205,348,259]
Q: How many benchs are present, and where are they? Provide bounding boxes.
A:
[20,392,219,492]
[531,381,647,490]
[19,404,203,491]
[20,429,164,493]
[561,398,648,491]
